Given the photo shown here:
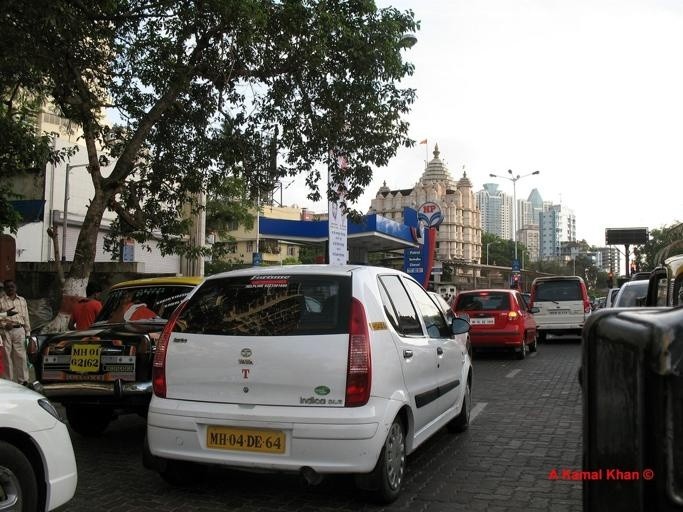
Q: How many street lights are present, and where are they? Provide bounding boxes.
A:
[491,169,542,269]
[486,242,503,269]
[63,160,111,261]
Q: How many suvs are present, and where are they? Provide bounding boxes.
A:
[30,276,215,436]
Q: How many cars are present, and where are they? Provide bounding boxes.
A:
[425,289,541,359]
[0,380,79,511]
[574,254,682,511]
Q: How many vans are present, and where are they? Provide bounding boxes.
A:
[530,276,593,339]
[140,266,473,504]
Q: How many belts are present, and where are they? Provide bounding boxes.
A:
[5,324,24,328]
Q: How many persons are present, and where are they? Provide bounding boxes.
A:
[0,306,20,374]
[0,279,30,385]
[68,281,102,331]
